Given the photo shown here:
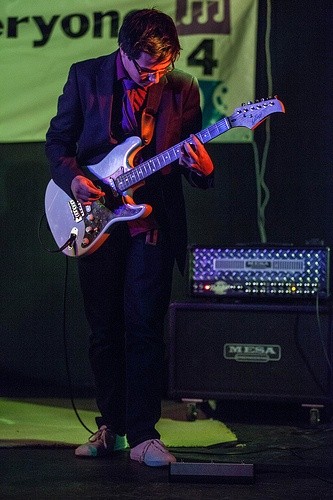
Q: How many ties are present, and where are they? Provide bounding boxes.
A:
[122,79,138,135]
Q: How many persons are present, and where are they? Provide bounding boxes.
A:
[46,8,216,467]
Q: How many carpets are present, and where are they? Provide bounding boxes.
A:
[0,399,239,448]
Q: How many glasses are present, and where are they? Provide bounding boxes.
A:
[131,56,174,76]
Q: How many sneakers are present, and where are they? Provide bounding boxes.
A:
[75,424,115,457]
[130,439,176,467]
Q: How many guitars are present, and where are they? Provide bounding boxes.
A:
[45,96,285,258]
